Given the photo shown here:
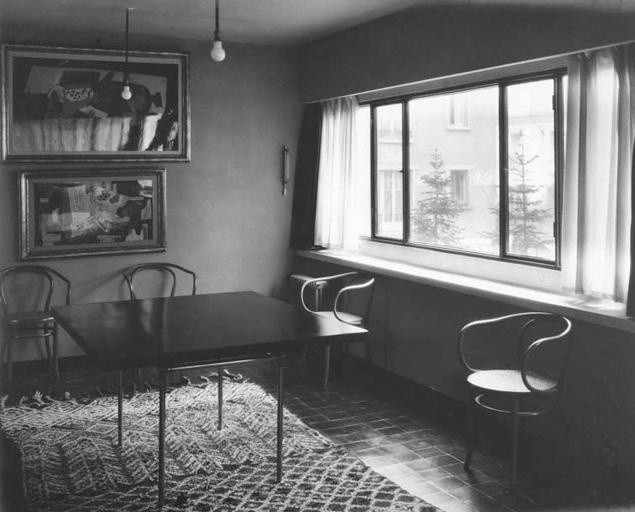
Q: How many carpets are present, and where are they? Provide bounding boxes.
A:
[0,366,440,512]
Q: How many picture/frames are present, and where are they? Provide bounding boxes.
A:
[0,40,195,166]
[12,163,170,264]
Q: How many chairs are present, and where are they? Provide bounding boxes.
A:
[0,261,74,389]
[458,304,575,504]
[279,269,379,391]
[119,261,200,303]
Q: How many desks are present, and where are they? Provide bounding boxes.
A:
[44,289,374,512]
